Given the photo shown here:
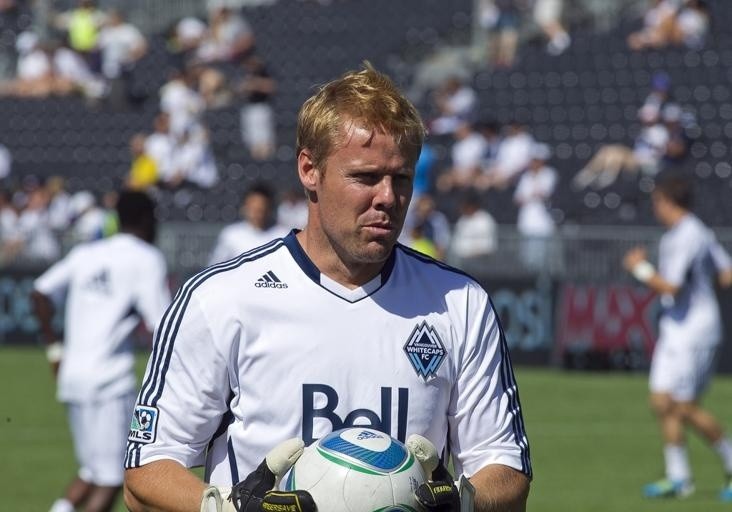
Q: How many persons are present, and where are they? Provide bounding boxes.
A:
[617,174,732,506]
[571,74,696,194]
[404,72,560,269]
[29,188,177,511]
[0,0,308,269]
[119,59,535,512]
[466,2,711,68]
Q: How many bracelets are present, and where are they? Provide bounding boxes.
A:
[630,260,656,285]
[46,340,67,365]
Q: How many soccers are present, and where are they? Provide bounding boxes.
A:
[279,426,429,512]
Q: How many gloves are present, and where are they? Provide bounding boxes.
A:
[200,438,317,512]
[406,434,476,512]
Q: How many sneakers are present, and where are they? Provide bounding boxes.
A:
[643,475,697,499]
[719,471,732,503]
